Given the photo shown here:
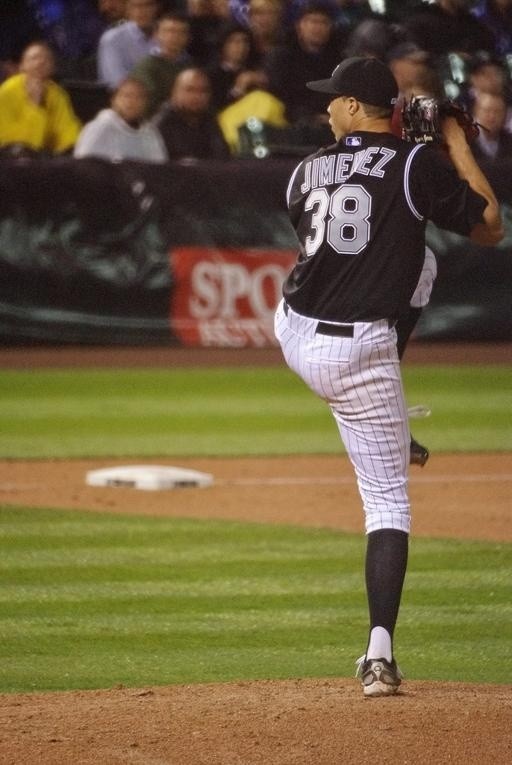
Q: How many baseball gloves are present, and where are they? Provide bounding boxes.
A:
[401,94,479,147]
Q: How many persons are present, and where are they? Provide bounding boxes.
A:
[272,50,505,698]
[0,1,512,246]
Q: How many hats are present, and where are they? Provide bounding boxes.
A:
[305,57,398,108]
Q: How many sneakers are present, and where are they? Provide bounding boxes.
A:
[355,654,404,697]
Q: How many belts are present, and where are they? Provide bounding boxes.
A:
[283,300,398,337]
